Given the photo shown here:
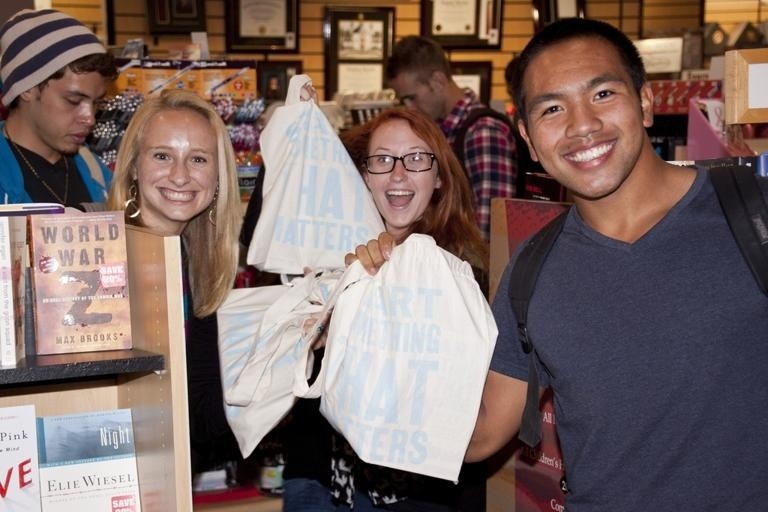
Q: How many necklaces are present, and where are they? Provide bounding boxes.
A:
[7,133,81,206]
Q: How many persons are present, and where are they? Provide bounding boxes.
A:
[104,88,246,479]
[382,33,524,302]
[240,73,488,511]
[346,17,767,512]
[0,9,112,212]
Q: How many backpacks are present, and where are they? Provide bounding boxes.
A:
[214,280,329,460]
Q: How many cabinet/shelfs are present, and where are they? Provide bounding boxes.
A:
[0,220,195,512]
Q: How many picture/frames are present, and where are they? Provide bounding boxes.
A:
[322,5,394,100]
[449,59,496,103]
[418,0,506,50]
[147,1,205,37]
[222,0,300,52]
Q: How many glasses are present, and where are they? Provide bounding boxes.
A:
[359,151,434,175]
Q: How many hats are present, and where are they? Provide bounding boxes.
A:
[1,8,107,107]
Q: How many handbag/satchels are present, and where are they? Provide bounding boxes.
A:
[320,231,498,486]
[246,72,387,273]
